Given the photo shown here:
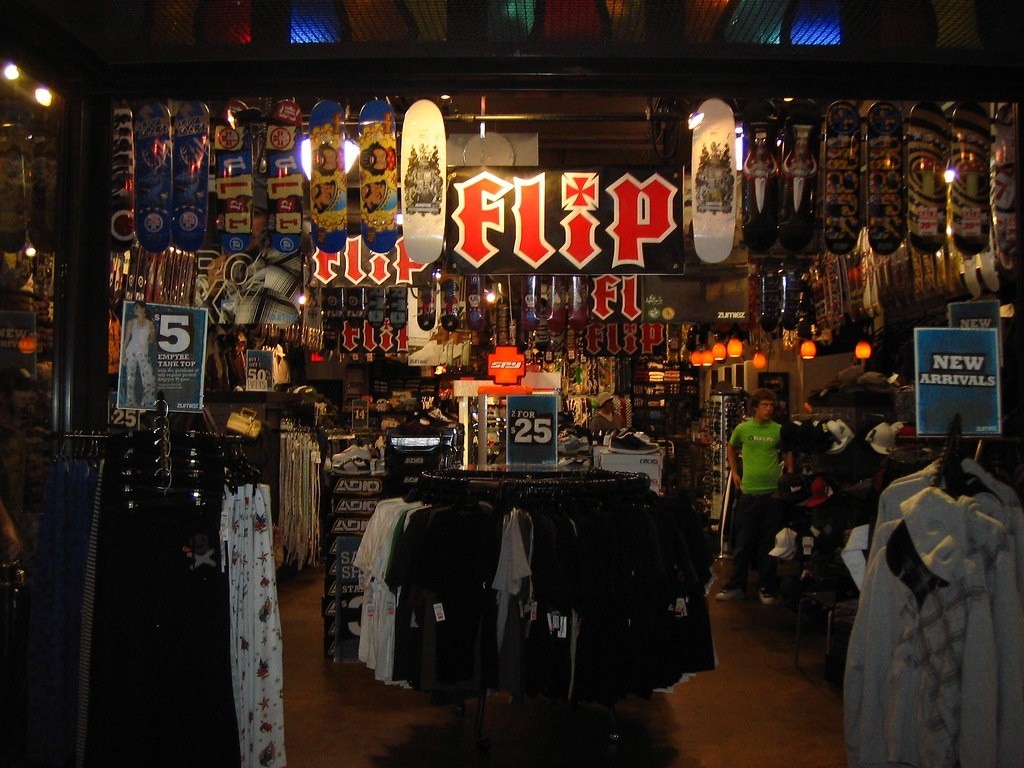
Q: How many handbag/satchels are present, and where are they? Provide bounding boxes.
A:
[226,407,262,440]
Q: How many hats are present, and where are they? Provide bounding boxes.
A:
[773,421,923,455]
[769,527,797,560]
[806,477,833,507]
[597,391,614,406]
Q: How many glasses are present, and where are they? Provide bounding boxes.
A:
[703,398,745,510]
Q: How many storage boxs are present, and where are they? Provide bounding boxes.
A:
[597,453,664,485]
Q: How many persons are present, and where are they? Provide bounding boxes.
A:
[123,301,158,409]
[0,498,22,562]
[715,388,794,605]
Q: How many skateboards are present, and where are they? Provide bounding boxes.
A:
[908,101,947,255]
[358,99,399,253]
[399,98,447,267]
[692,98,738,264]
[866,102,905,257]
[951,102,991,255]
[743,98,782,253]
[264,98,302,251]
[783,99,821,253]
[0,60,1024,430]
[826,99,863,255]
[307,100,348,252]
[994,104,1016,271]
[134,102,171,251]
[174,100,208,252]
[105,105,135,252]
[214,100,252,251]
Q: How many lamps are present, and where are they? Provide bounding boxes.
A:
[753,353,766,368]
[855,341,871,358]
[691,351,702,366]
[712,343,726,360]
[702,350,714,366]
[801,340,817,359]
[727,338,743,357]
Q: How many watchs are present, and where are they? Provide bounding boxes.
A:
[589,392,620,434]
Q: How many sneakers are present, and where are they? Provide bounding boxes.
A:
[331,443,371,462]
[610,432,658,455]
[332,456,371,475]
[715,585,745,600]
[759,588,777,605]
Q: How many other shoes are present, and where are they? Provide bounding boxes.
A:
[395,405,458,436]
[557,427,589,453]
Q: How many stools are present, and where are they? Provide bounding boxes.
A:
[794,592,853,683]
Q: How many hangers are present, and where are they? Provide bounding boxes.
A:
[117,398,208,509]
[52,429,102,471]
[928,422,963,493]
[403,465,648,508]
